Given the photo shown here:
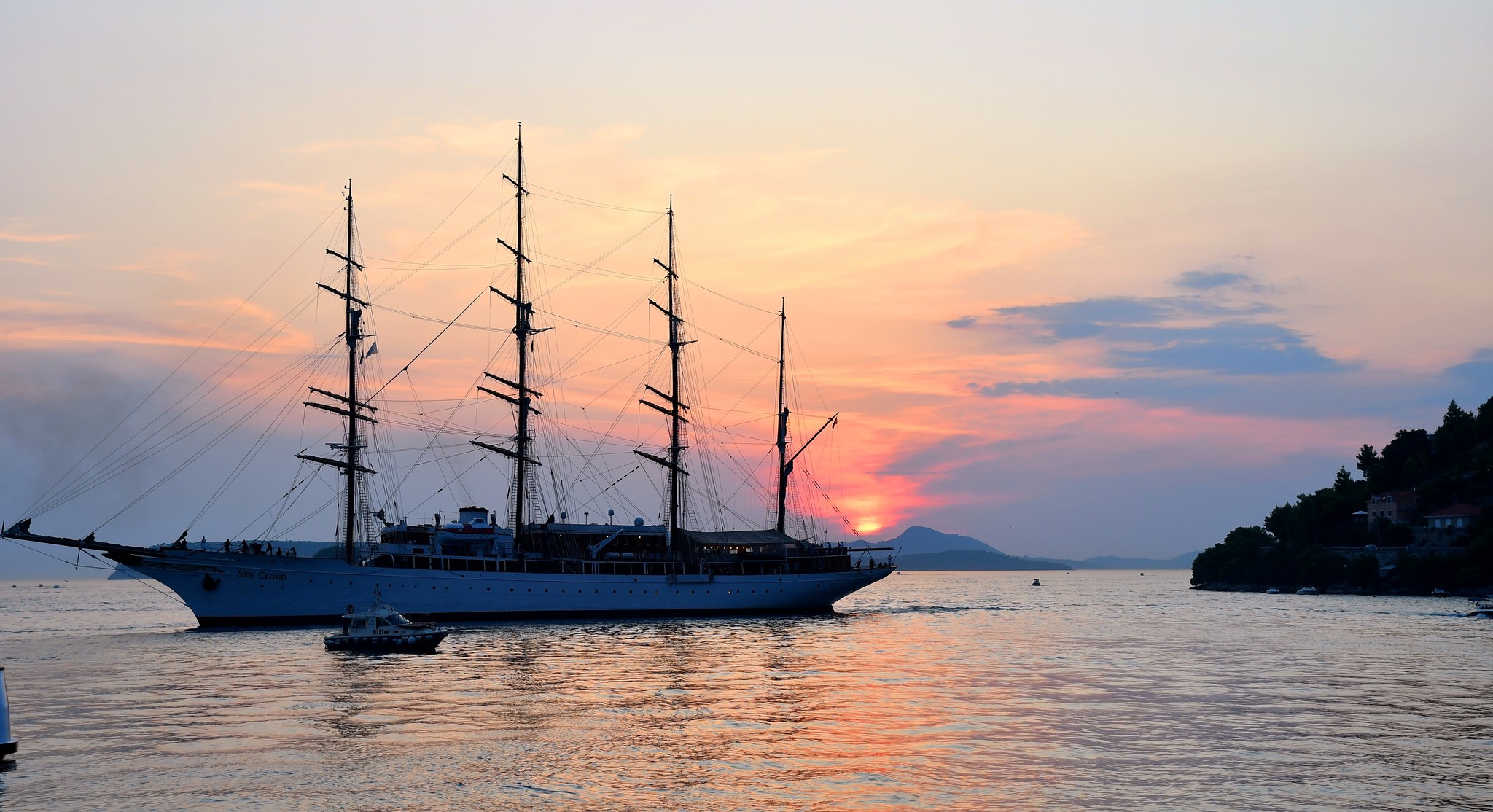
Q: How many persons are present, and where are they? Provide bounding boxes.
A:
[182,536,297,557]
[797,541,893,569]
[408,538,421,545]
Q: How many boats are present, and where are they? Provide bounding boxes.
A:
[1432,585,1451,597]
[1466,597,1493,617]
[1032,579,1041,586]
[1266,587,1280,594]
[1296,586,1319,595]
[323,580,452,654]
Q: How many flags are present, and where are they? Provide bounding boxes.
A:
[360,340,377,365]
[832,416,837,430]
[530,340,534,352]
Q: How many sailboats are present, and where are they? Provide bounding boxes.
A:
[0,118,905,629]
